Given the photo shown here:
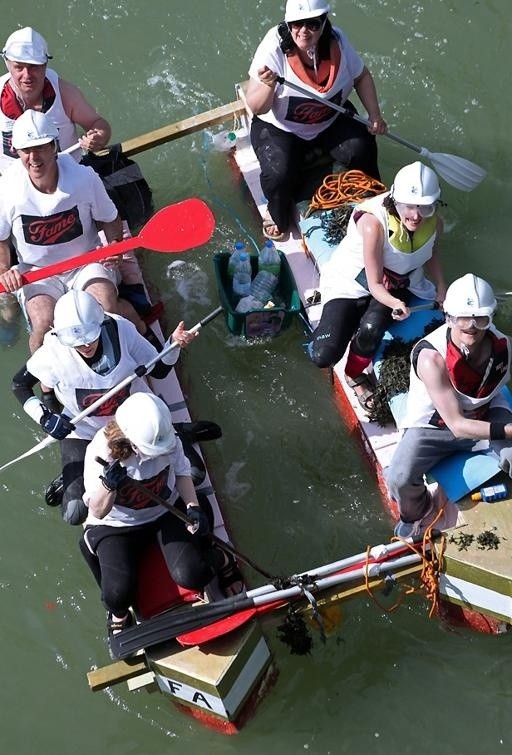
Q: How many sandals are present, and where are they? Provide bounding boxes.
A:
[107,609,133,636]
[344,373,385,413]
[262,205,285,240]
[213,542,244,598]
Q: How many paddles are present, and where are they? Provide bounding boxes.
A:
[175,538,433,647]
[0,198,216,293]
[152,532,445,616]
[275,75,486,194]
[0,305,228,476]
[110,548,438,659]
[92,455,343,638]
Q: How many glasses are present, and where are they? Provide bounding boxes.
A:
[394,201,439,218]
[447,314,493,331]
[288,19,321,31]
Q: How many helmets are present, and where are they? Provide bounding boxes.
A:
[0,27,53,65]
[443,273,497,317]
[389,160,441,206]
[53,289,104,347]
[284,0,330,32]
[11,109,62,152]
[115,391,176,456]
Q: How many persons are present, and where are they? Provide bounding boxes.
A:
[306,158,446,412]
[0,25,112,162]
[79,391,248,638]
[0,109,163,414]
[386,271,512,539]
[246,0,388,242]
[8,289,201,526]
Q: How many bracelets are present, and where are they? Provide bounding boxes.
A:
[489,421,509,439]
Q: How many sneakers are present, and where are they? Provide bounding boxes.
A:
[44,472,64,506]
[394,491,434,539]
[136,323,164,353]
[173,421,222,444]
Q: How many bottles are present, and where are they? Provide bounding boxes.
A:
[213,132,237,153]
[470,483,509,503]
[228,239,282,316]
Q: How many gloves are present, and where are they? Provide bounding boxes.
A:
[103,458,127,491]
[185,506,209,536]
[40,413,76,440]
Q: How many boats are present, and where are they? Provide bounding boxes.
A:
[0,148,274,737]
[224,79,512,628]
